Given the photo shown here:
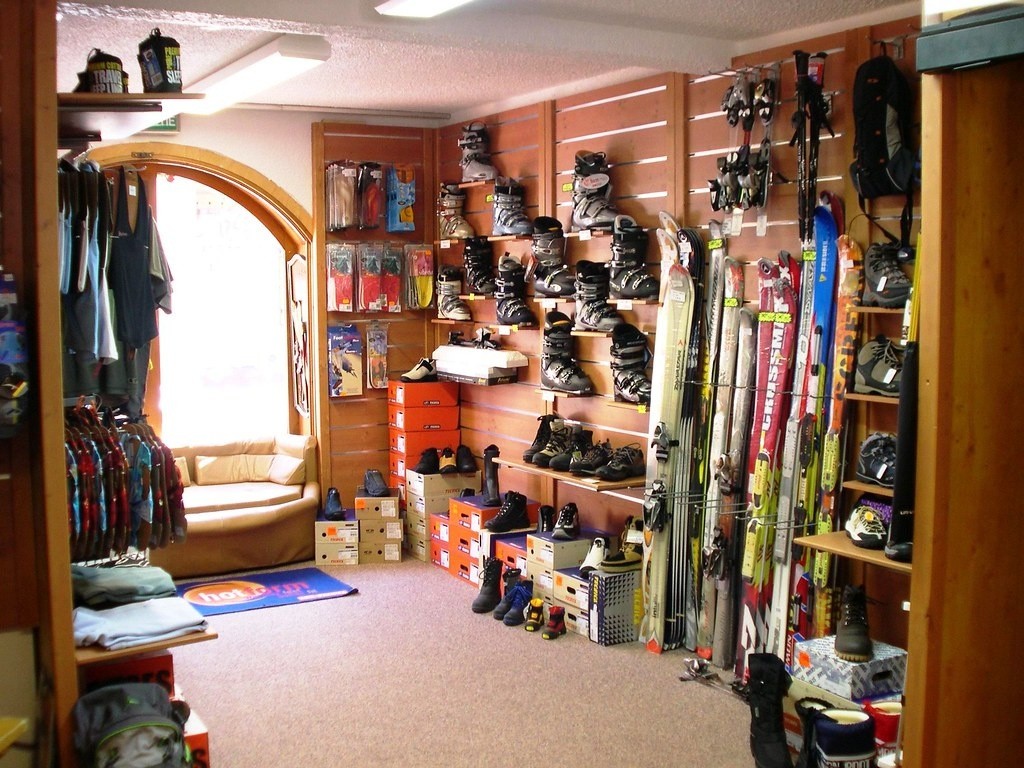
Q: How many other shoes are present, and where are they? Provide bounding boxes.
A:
[325,486,344,519]
[577,536,613,580]
[414,444,479,475]
[363,467,389,498]
[447,488,476,516]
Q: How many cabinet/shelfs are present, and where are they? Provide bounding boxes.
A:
[57,90,220,664]
[431,178,661,494]
[793,306,912,573]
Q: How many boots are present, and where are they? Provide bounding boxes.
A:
[471,555,504,613]
[434,121,657,407]
[599,515,645,572]
[542,605,567,640]
[481,442,502,507]
[745,651,882,768]
[535,505,557,534]
[492,568,523,620]
[524,598,544,632]
[835,584,886,661]
[503,579,536,626]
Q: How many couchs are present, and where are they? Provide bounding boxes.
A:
[147,430,318,581]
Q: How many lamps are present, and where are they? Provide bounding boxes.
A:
[179,35,332,117]
[375,0,475,18]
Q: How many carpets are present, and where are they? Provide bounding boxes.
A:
[175,563,357,618]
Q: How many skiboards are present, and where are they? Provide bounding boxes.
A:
[636,189,863,680]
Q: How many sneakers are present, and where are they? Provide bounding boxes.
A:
[844,504,889,548]
[853,430,897,490]
[522,413,646,483]
[859,241,914,309]
[853,334,902,400]
[550,501,580,541]
[484,490,530,532]
[399,355,438,381]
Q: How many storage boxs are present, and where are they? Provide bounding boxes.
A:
[314,385,643,647]
[783,635,903,752]
[84,647,212,768]
